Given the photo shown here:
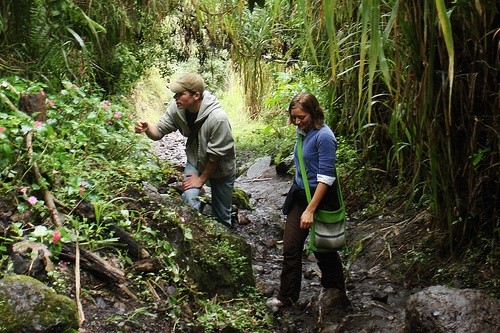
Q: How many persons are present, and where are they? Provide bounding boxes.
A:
[265,91,347,313]
[134,72,236,231]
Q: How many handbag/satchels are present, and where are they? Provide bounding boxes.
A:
[305,207,349,255]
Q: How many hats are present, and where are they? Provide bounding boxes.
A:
[166,72,205,94]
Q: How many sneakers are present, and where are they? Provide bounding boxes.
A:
[307,284,347,313]
[266,297,293,308]
